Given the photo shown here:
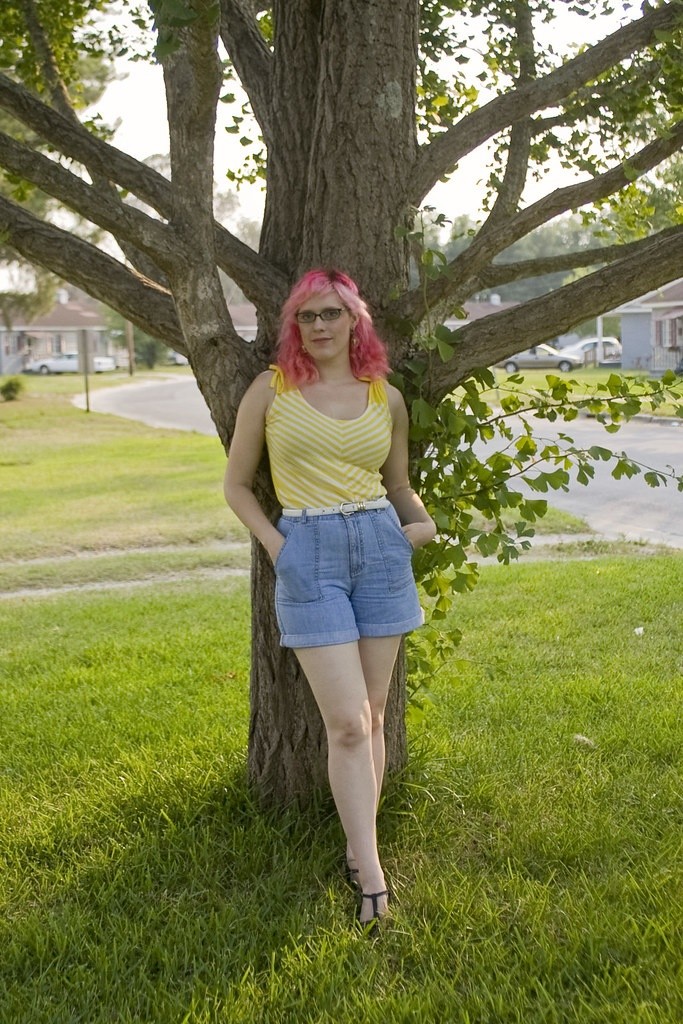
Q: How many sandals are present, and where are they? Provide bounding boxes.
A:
[359,882,390,934]
[347,868,362,892]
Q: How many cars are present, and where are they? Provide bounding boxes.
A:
[495,344,584,374]
[30,351,115,375]
[563,336,622,361]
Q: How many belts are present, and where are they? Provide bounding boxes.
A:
[282,495,390,518]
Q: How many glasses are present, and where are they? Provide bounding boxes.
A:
[295,307,349,323]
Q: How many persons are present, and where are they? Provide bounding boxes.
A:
[223,269,437,930]
